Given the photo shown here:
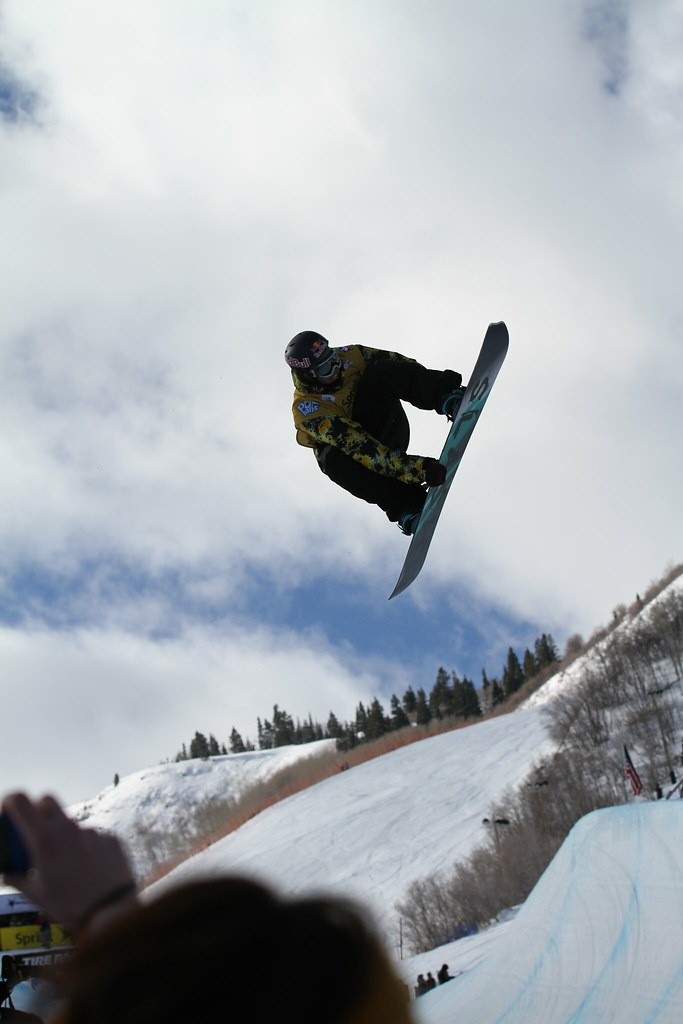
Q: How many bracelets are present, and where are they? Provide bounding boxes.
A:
[82,881,136,924]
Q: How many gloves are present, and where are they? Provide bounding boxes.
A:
[421,457,446,487]
[439,369,462,394]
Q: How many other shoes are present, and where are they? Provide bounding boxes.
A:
[443,391,467,421]
[399,511,420,534]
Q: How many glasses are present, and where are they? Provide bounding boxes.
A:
[314,351,341,379]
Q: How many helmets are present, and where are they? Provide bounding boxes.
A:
[284,330,328,371]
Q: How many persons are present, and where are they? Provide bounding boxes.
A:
[0,793,412,1024]
[655,784,662,798]
[285,331,466,537]
[417,964,454,994]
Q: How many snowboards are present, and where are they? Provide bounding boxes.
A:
[390,321,510,598]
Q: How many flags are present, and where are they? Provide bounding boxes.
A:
[624,745,643,795]
